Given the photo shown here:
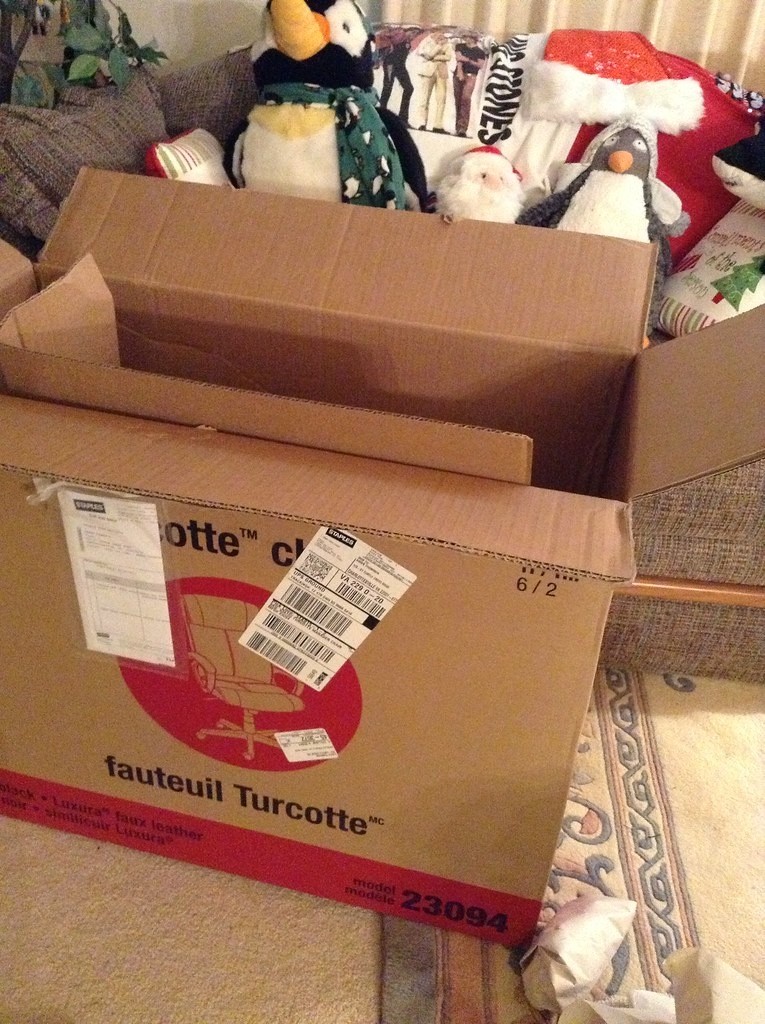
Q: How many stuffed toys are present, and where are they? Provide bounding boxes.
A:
[711,134,765,211]
[516,115,691,343]
[222,0,427,213]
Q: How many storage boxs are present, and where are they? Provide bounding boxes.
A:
[0,164,765,955]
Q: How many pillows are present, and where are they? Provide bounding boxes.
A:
[145,129,233,189]
[155,44,264,170]
[654,198,765,339]
[3,60,173,262]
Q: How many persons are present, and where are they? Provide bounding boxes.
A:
[440,146,524,223]
